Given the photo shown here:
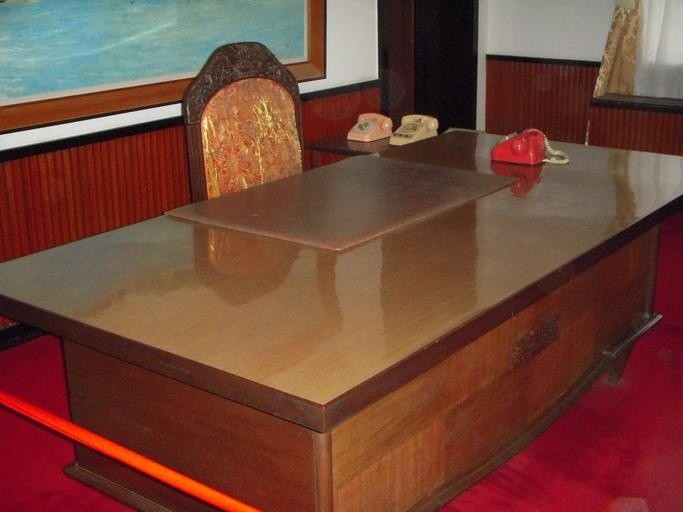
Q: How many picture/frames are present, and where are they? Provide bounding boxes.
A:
[0,0,328,134]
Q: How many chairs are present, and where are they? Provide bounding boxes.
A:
[181,41,304,203]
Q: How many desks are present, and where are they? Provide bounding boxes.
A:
[0,129,683,511]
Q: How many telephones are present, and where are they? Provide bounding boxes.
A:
[490,128,548,165]
[389,114,439,146]
[346,113,393,142]
